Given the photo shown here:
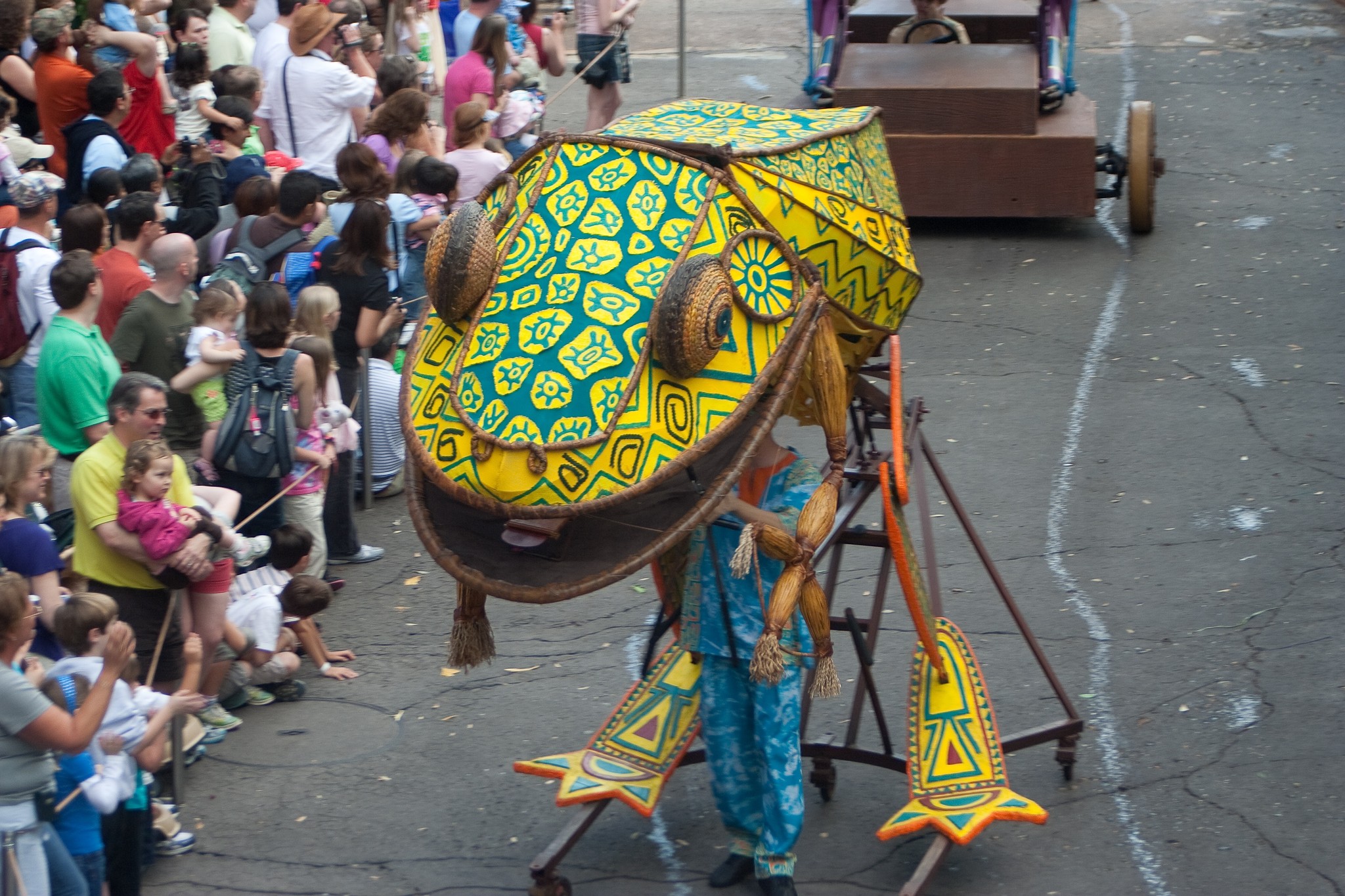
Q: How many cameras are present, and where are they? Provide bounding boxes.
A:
[181,136,198,154]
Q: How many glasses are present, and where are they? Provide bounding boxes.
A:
[17,595,44,622]
[128,408,173,419]
[405,55,415,62]
[364,41,385,52]
[153,219,168,227]
[124,86,136,96]
[423,116,430,123]
[26,469,50,478]
[95,267,103,277]
[354,196,389,219]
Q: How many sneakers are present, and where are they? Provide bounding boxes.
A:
[237,682,274,706]
[197,702,242,728]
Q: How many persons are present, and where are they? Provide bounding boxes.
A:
[0,0,633,895]
[886,0,974,45]
[678,416,822,896]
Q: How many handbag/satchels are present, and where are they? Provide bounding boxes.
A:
[573,59,608,90]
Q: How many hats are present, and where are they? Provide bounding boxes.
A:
[3,137,54,169]
[7,171,66,207]
[507,0,530,7]
[30,4,78,43]
[494,90,536,137]
[264,150,303,172]
[288,2,349,56]
[455,108,500,132]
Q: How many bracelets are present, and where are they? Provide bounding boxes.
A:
[318,661,331,675]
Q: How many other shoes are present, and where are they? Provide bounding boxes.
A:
[707,854,755,890]
[235,535,270,567]
[181,723,226,765]
[322,570,346,591]
[756,875,798,896]
[192,458,217,485]
[275,679,308,702]
[148,798,177,819]
[152,831,197,854]
[329,545,386,564]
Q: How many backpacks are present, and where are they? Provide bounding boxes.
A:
[0,227,54,368]
[211,341,301,482]
[269,235,338,308]
[199,214,306,296]
